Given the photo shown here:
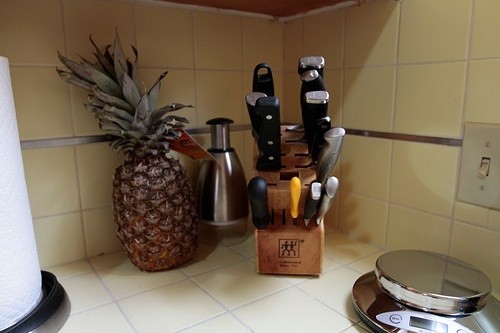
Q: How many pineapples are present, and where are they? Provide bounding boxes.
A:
[55,26,197,273]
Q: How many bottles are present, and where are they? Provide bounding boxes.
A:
[193,118,249,247]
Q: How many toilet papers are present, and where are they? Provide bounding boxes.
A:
[0,55,45,331]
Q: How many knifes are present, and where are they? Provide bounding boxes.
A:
[298,55,332,164]
[304,182,322,227]
[246,61,282,170]
[316,176,339,226]
[289,176,302,226]
[312,127,346,188]
[248,177,272,229]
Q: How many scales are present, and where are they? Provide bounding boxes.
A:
[351,250,500,333]
